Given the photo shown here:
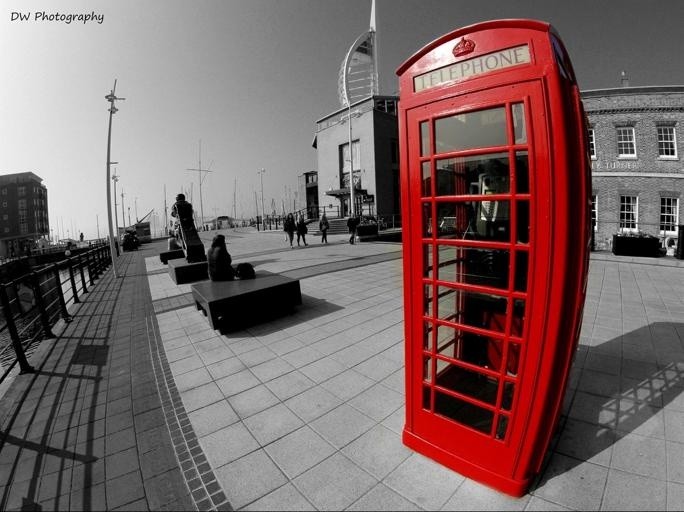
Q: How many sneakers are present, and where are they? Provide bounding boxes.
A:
[290,243,309,249]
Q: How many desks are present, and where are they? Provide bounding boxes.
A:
[159,249,184,264]
[191,270,302,336]
[168,259,208,284]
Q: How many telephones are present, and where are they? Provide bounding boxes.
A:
[469,171,509,239]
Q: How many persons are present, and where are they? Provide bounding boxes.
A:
[347,213,356,244]
[171,194,187,218]
[318,214,329,243]
[167,235,182,250]
[167,220,174,238]
[296,215,308,248]
[206,235,238,281]
[284,213,297,249]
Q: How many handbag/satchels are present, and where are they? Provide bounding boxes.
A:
[284,231,288,241]
[235,262,256,280]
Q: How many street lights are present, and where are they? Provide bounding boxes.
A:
[339,99,362,214]
[105,78,131,279]
[256,168,265,225]
